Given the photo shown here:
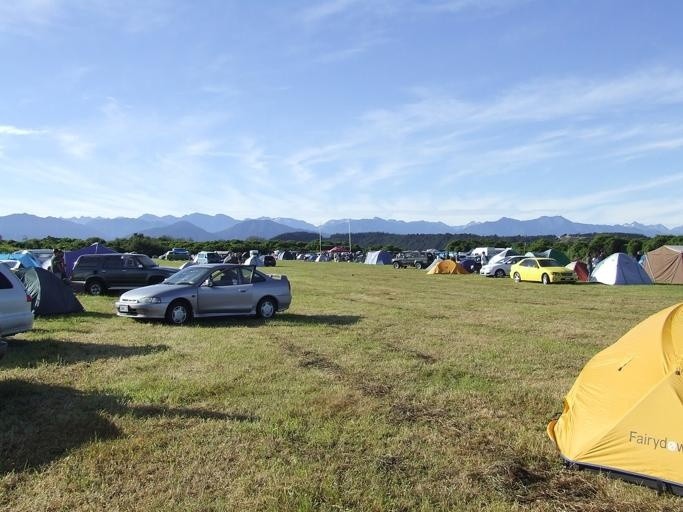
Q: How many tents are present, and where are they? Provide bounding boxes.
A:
[525,248,571,267]
[0,261,33,336]
[423,255,443,272]
[0,253,40,269]
[12,265,86,318]
[178,261,197,271]
[547,301,682,501]
[565,260,589,281]
[243,255,263,266]
[640,244,683,285]
[590,252,652,285]
[426,260,469,275]
[222,255,238,264]
[363,250,394,265]
[488,249,520,264]
[63,241,118,291]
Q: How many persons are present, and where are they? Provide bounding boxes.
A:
[635,250,646,264]
[237,252,241,265]
[334,252,340,263]
[54,251,63,268]
[481,251,487,266]
[346,251,352,262]
[51,249,62,279]
[591,255,599,271]
[473,253,481,274]
[598,248,607,263]
